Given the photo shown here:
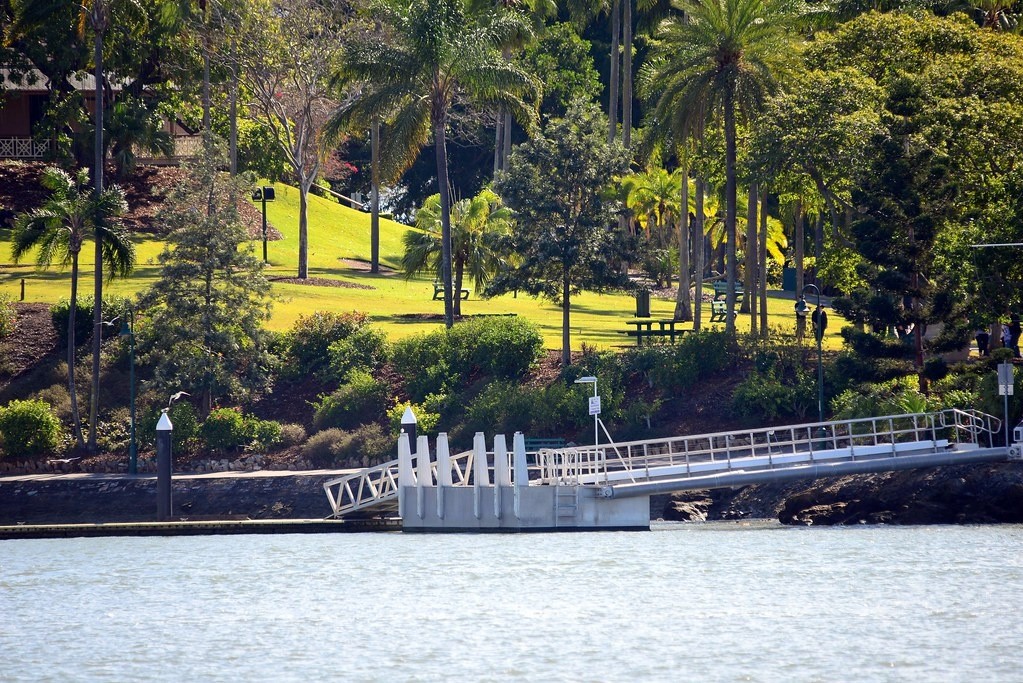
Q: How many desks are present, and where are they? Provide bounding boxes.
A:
[625,317,685,349]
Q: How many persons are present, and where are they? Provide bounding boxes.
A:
[812,303,827,347]
[975,312,1022,358]
[795,294,806,337]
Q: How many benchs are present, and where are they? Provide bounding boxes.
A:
[708,300,739,323]
[616,328,695,347]
[712,281,752,301]
[431,277,474,300]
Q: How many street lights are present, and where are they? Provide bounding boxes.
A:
[116,307,138,477]
[574,373,601,488]
[793,283,827,454]
